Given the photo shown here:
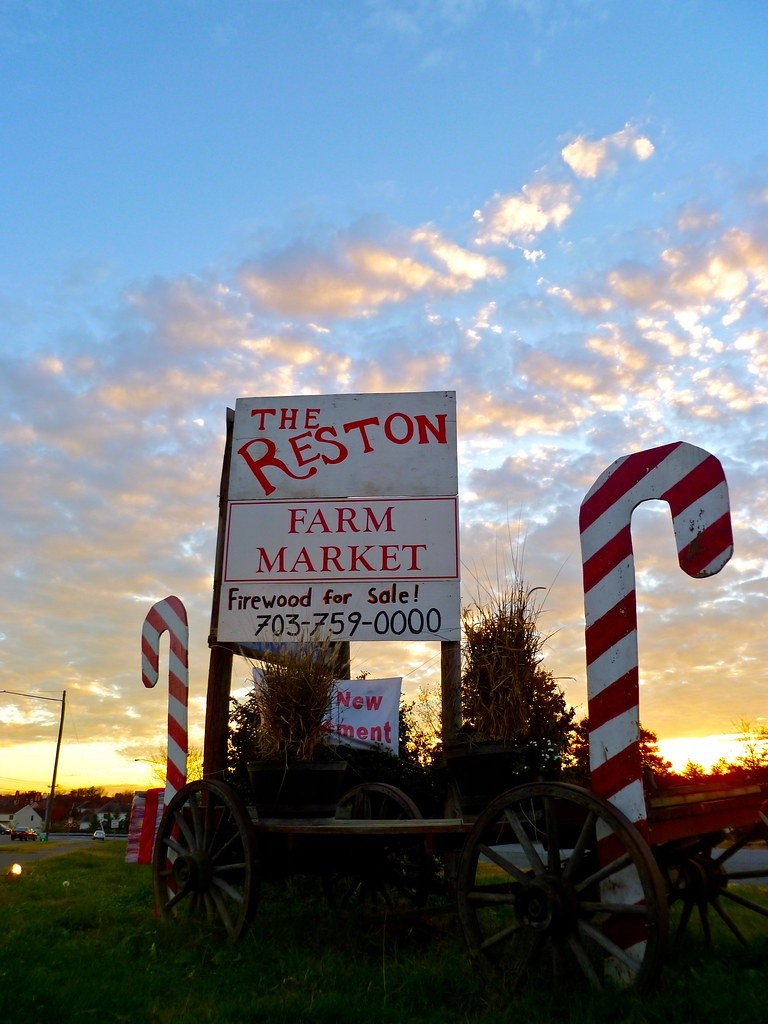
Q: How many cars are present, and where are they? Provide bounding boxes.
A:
[11,827,38,841]
[0,824,12,835]
[92,830,107,840]
